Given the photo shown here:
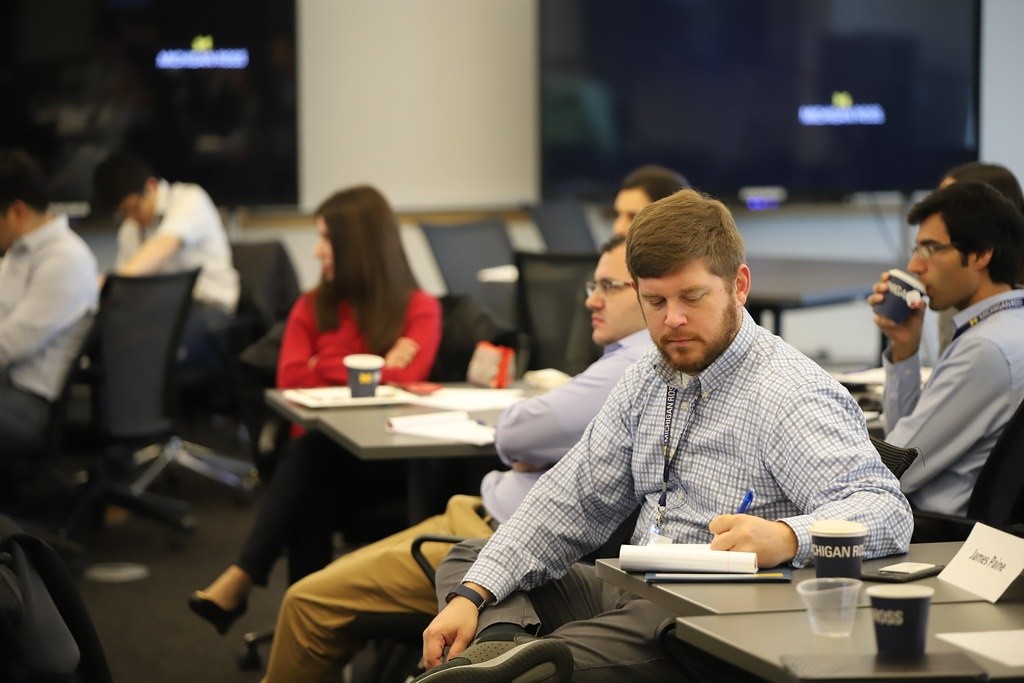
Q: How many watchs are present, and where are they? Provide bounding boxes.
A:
[445,584,487,616]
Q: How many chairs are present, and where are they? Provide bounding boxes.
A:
[419,198,606,377]
[0,240,302,683]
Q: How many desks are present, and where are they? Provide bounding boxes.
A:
[262,380,527,531]
[594,541,1024,683]
[742,257,910,339]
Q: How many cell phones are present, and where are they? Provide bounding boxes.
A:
[861,563,945,584]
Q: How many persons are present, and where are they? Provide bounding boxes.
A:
[0,159,100,456]
[259,234,655,683]
[866,161,1024,518]
[406,189,915,683]
[187,185,444,636]
[99,161,240,400]
[611,163,687,241]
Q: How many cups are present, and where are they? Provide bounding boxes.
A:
[796,577,862,637]
[866,584,935,662]
[809,520,870,596]
[872,267,926,325]
[343,353,386,398]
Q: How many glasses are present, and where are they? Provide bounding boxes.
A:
[585,280,637,298]
[911,244,952,258]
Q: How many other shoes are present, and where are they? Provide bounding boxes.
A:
[189,591,240,636]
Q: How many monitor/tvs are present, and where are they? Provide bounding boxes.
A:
[534,0,984,206]
[0,0,301,212]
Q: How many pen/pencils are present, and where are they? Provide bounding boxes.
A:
[737,489,756,514]
